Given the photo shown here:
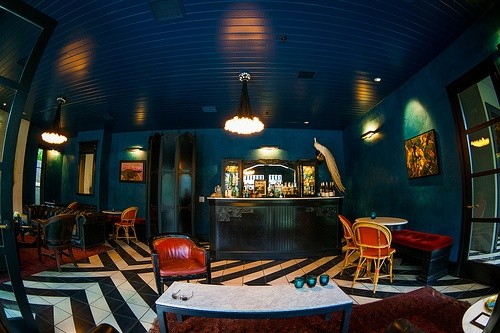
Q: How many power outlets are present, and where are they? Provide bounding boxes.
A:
[198,196,204,202]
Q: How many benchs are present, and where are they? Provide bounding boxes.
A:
[390,229,452,283]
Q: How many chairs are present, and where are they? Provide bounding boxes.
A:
[36,214,78,273]
[148,232,211,298]
[115,207,139,244]
[349,221,396,293]
[66,201,78,210]
[338,215,375,276]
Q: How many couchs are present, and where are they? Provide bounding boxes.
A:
[23,204,107,251]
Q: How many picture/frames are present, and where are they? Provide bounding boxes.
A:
[403,128,441,180]
[119,159,146,184]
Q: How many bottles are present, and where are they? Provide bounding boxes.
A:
[320,181,336,197]
[224,181,298,198]
[214,184,223,198]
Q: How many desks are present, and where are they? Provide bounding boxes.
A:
[102,211,123,239]
[355,217,409,278]
[0,225,31,266]
[155,281,353,333]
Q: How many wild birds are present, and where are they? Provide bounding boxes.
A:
[314,138,346,196]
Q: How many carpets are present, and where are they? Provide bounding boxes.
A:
[146,285,472,333]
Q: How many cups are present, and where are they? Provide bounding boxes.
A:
[306,276,317,288]
[294,277,304,288]
[320,274,330,285]
[370,211,376,219]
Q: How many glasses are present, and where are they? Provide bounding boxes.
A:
[172,289,193,301]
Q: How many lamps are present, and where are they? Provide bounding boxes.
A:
[41,97,68,144]
[223,72,265,135]
